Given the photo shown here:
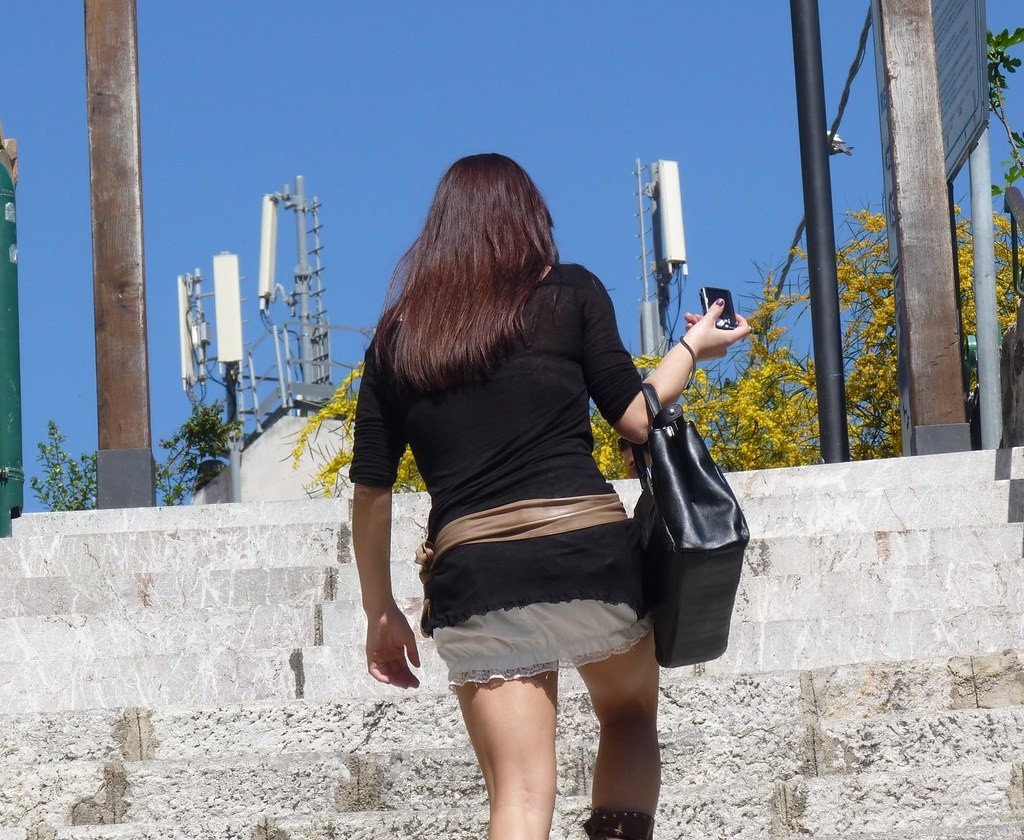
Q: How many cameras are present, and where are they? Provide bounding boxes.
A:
[700,287,737,330]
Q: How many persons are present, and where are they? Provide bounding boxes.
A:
[348,153,749,840]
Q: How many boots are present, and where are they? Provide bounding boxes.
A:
[582,808,656,840]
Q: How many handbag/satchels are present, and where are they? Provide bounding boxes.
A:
[629,383,749,667]
